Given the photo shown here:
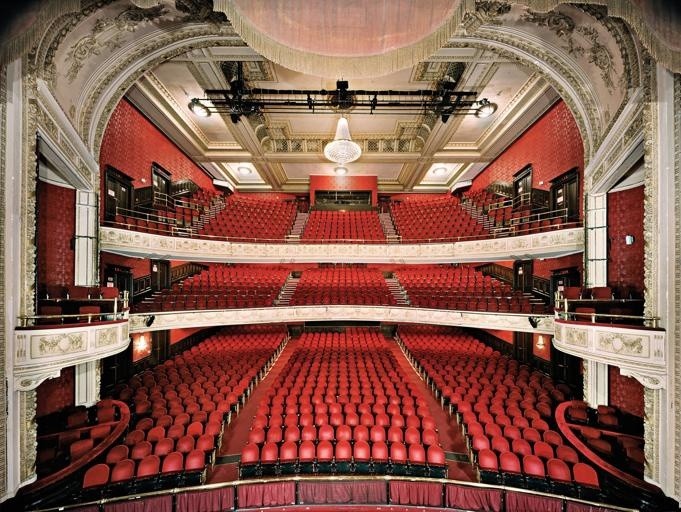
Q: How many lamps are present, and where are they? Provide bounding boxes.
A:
[324,116,361,165]
